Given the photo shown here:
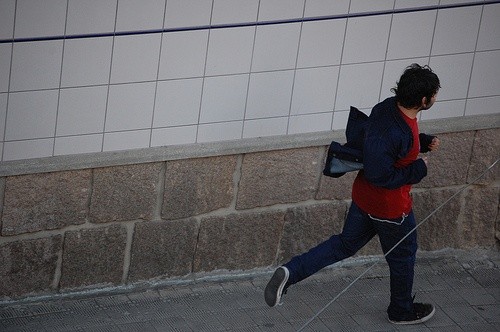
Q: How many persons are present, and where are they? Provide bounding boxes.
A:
[264,62,442,325]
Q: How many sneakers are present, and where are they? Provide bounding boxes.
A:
[386,301,436,325]
[263,265,289,308]
